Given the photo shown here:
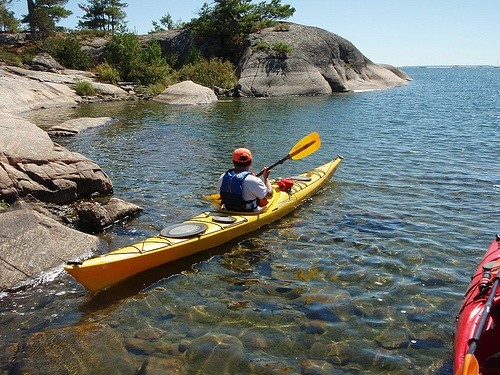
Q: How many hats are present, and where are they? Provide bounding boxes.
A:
[232,148,252,162]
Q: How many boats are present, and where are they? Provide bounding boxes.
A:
[453,233,500,375]
[62,152,343,292]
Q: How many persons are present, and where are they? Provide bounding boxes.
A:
[217,147,273,213]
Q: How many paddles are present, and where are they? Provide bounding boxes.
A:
[205,131,321,206]
[454,270,500,375]
